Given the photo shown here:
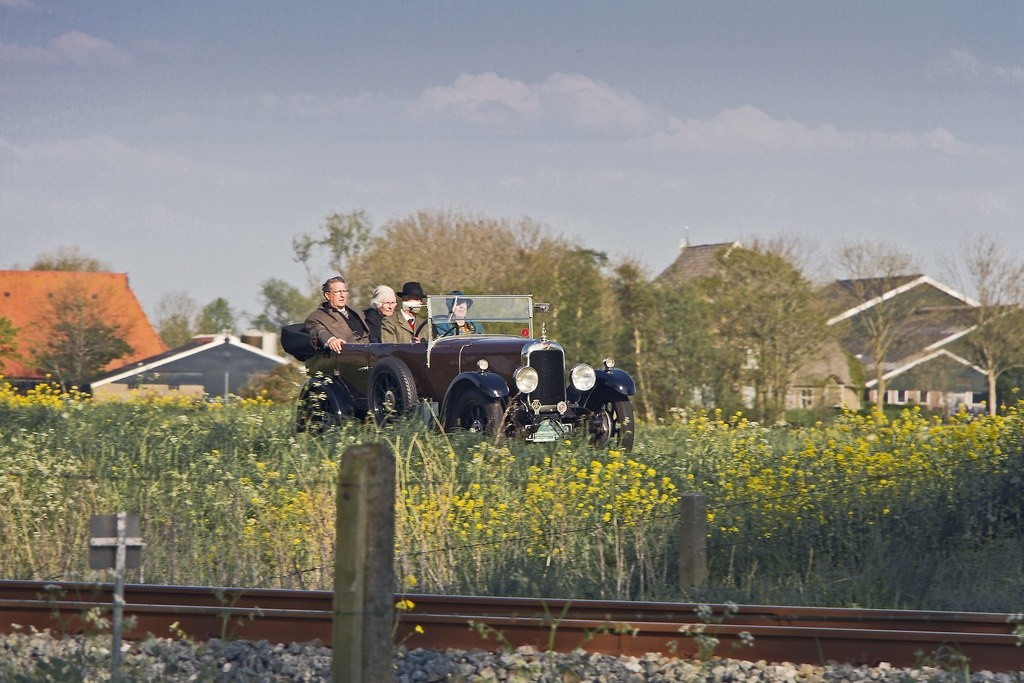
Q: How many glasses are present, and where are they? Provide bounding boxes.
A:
[381,301,397,307]
[324,289,349,296]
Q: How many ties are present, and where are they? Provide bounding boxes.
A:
[409,319,414,323]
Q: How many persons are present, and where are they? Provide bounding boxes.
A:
[365,284,398,343]
[435,290,485,336]
[381,282,456,344]
[304,276,371,355]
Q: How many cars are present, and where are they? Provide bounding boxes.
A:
[280,294,637,453]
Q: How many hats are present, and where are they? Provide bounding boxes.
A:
[396,282,426,298]
[446,291,473,313]
